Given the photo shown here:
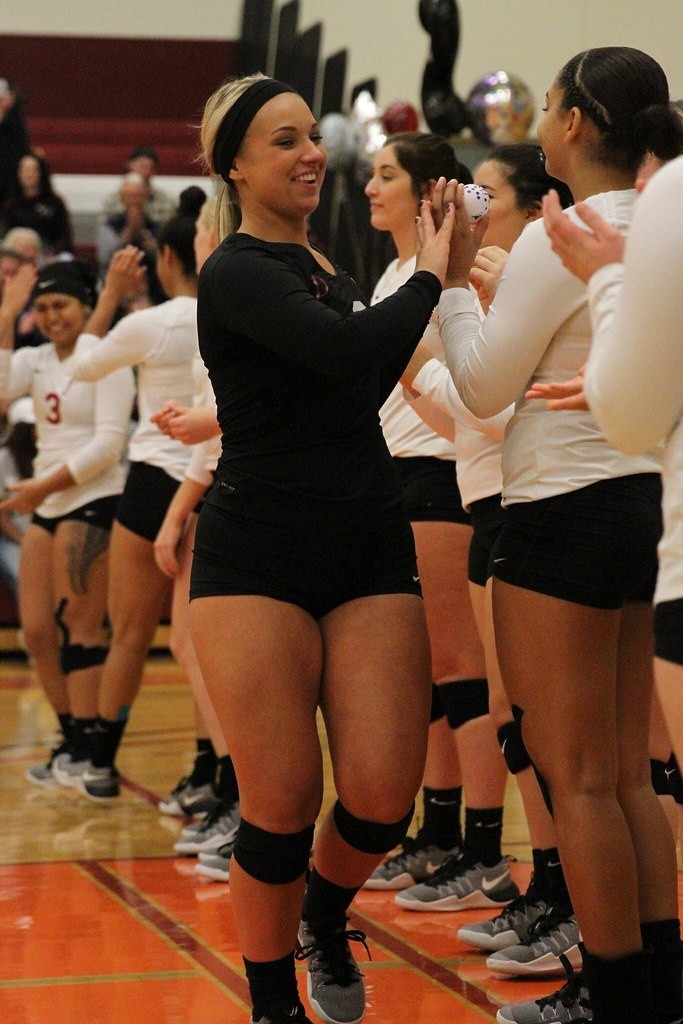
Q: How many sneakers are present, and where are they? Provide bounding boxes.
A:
[158,777,240,882]
[495,940,680,1024]
[394,852,520,912]
[27,752,122,802]
[294,894,366,1024]
[457,877,583,976]
[360,828,459,890]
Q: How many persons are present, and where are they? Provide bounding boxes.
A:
[428,44,683,1024]
[0,61,683,972]
[189,72,456,1024]
